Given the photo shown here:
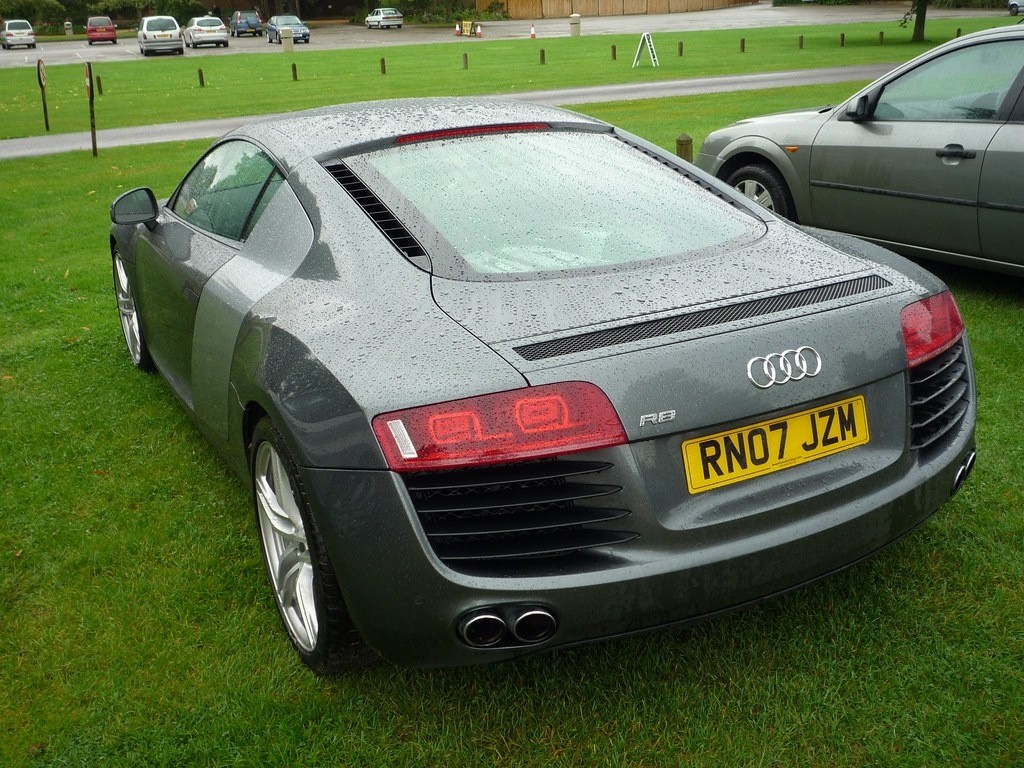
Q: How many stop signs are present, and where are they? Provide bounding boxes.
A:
[85,62,94,101]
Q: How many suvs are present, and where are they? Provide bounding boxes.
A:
[227,10,263,37]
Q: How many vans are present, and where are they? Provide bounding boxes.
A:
[137,15,184,57]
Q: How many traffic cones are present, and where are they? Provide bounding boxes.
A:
[454,21,461,36]
[475,23,482,38]
[530,23,536,38]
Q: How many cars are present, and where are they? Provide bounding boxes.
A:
[692,24,1024,283]
[182,15,228,48]
[0,18,36,50]
[83,15,118,45]
[1007,0,1024,17]
[265,13,310,44]
[364,7,404,30]
[107,95,981,679]
